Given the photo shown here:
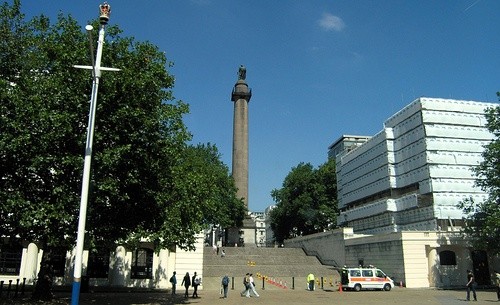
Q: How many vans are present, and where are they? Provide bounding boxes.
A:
[342,267,395,292]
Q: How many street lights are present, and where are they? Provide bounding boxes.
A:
[70,1,123,305]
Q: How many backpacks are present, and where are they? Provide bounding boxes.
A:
[243,278,246,284]
[170,277,173,282]
[222,279,227,285]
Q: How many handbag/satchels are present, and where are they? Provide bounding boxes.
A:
[220,287,223,295]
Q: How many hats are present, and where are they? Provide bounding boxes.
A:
[246,273,249,275]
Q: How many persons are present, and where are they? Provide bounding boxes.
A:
[172,272,176,295]
[221,250,225,260]
[221,273,230,298]
[490,270,500,301]
[307,273,315,291]
[181,272,191,298]
[217,240,221,255]
[465,269,477,301]
[192,272,201,298]
[246,273,260,298]
[240,273,254,297]
